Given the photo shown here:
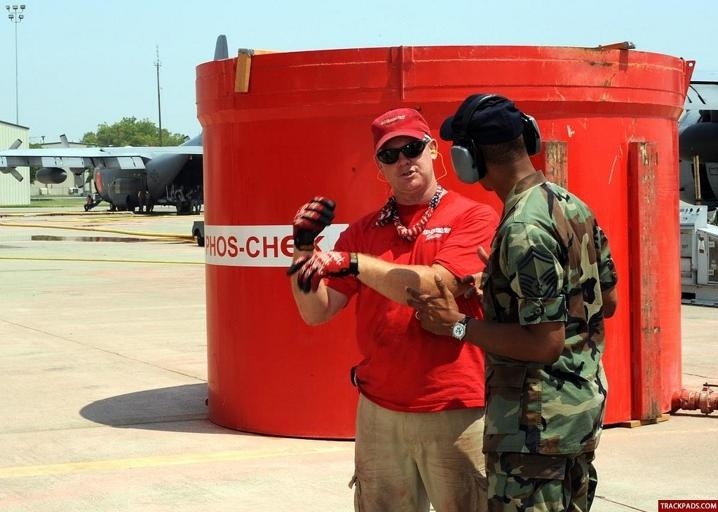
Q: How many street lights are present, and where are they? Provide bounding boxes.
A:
[154,42,164,144]
[7,3,25,123]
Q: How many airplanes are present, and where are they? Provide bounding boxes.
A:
[0,132,204,214]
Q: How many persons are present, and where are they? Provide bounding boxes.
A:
[403,93,621,512]
[285,108,500,512]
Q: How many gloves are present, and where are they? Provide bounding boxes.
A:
[285,250,358,293]
[293,195,335,248]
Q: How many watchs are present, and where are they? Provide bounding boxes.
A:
[451,314,473,342]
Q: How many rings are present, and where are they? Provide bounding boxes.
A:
[414,311,422,321]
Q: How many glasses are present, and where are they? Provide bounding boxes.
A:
[375,139,430,163]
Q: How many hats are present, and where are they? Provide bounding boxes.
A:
[370,108,432,154]
[439,92,529,145]
